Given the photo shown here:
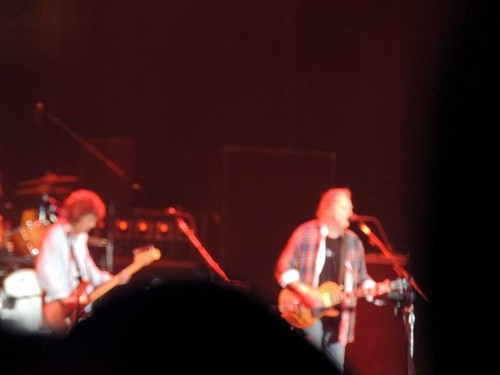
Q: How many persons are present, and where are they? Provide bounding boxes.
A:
[274,186,392,375]
[402,58,500,375]
[36,189,134,336]
[0,273,359,374]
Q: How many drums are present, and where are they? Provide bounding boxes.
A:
[6,220,45,262]
[0,215,11,250]
[0,267,51,336]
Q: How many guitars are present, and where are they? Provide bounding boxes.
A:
[42,247,162,338]
[277,277,411,330]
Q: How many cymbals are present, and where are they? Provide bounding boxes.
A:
[15,187,69,196]
[20,174,79,185]
